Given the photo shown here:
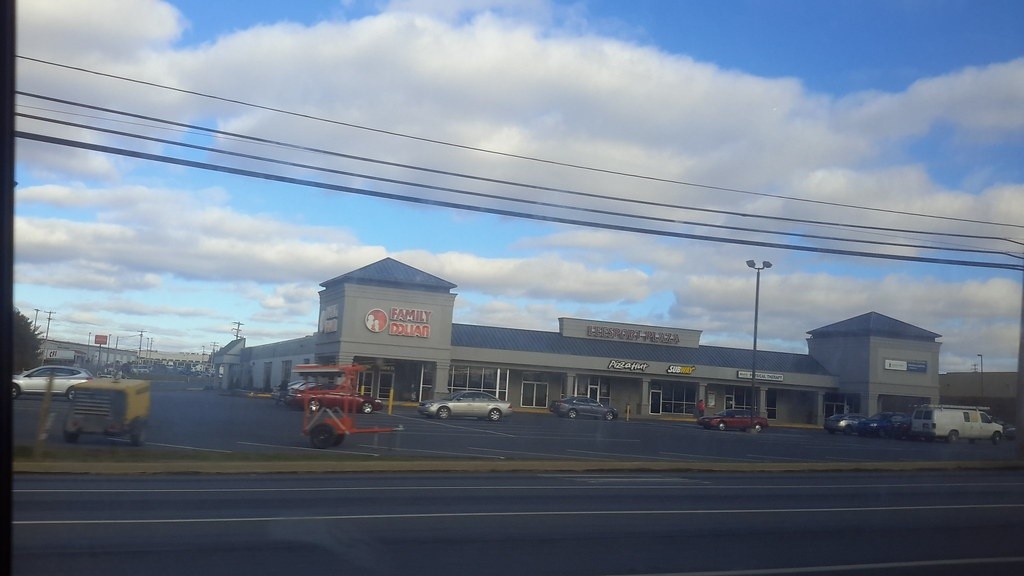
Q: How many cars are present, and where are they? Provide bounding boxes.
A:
[416,390,513,422]
[856,411,911,440]
[697,409,768,433]
[270,379,384,414]
[63,377,152,446]
[11,365,94,401]
[548,395,618,422]
[823,413,866,435]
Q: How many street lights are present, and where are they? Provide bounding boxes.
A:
[976,353,984,371]
[106,334,112,363]
[745,257,773,416]
[87,332,92,363]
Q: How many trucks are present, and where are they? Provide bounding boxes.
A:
[908,402,1003,445]
